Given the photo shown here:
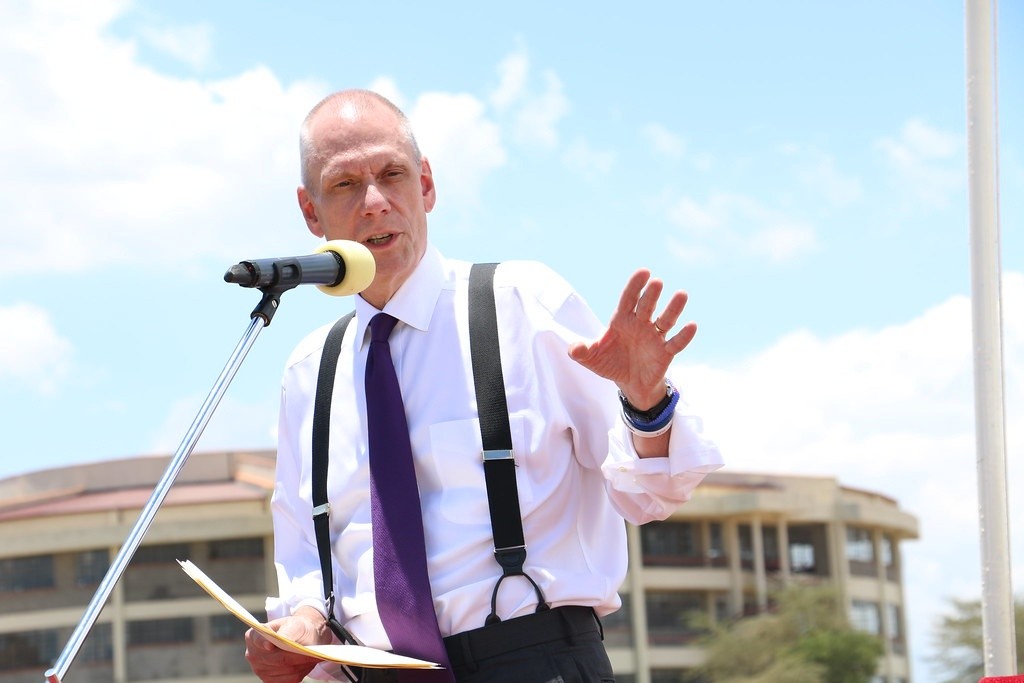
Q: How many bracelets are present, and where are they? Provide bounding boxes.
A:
[618,378,680,438]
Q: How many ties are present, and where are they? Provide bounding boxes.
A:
[365,313,451,683]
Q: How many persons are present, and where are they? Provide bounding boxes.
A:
[243,89,726,683]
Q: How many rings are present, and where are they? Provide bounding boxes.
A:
[654,322,667,335]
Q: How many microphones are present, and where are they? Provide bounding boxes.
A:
[224,239,376,297]
